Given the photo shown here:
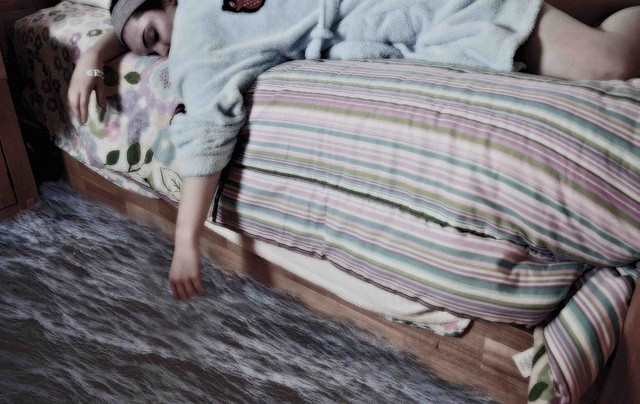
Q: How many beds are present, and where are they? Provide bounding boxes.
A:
[15,1,640,403]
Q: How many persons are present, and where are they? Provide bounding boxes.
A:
[67,1,640,302]
